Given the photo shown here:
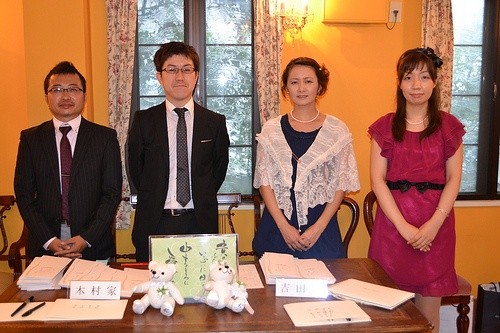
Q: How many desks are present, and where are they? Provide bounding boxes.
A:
[0,259,434,333]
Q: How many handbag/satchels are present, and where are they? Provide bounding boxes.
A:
[476,282,500,333]
[439,295,473,333]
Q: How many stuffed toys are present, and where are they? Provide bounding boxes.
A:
[132,260,184,317]
[203,260,248,312]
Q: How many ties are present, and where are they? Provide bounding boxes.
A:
[59,126,73,221]
[173,108,191,207]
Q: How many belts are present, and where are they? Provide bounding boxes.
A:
[162,209,195,216]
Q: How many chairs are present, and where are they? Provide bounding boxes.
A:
[363,191,472,333]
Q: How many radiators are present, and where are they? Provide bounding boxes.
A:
[218,213,227,233]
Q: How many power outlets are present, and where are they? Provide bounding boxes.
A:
[389,2,402,22]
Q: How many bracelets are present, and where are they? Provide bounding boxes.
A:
[436,207,449,215]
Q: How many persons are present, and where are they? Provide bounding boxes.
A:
[128,41,230,262]
[14,61,123,258]
[253,56,360,259]
[368,46,466,333]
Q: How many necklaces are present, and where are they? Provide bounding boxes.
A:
[405,115,428,125]
[291,108,319,123]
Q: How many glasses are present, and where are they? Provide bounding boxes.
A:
[47,87,84,95]
[162,66,196,74]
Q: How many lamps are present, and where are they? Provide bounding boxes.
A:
[273,0,308,46]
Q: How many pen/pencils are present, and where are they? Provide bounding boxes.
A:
[21,302,46,317]
[11,295,34,317]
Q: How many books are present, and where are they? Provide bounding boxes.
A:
[330,278,416,310]
